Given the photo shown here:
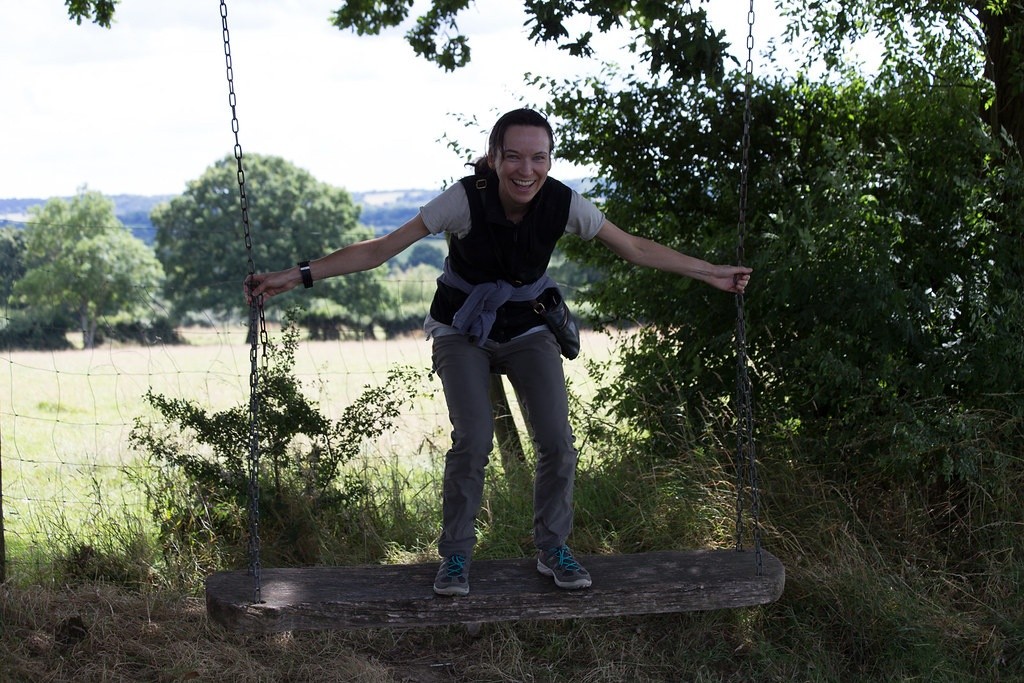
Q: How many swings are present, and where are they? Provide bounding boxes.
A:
[203,0,788,632]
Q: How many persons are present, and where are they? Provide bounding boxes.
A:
[244,107,750,594]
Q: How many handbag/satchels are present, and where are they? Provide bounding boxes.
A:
[534,288,580,360]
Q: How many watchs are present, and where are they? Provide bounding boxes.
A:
[297,259,314,288]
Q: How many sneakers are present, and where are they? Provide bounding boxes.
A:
[434,554,470,595]
[536,544,592,588]
